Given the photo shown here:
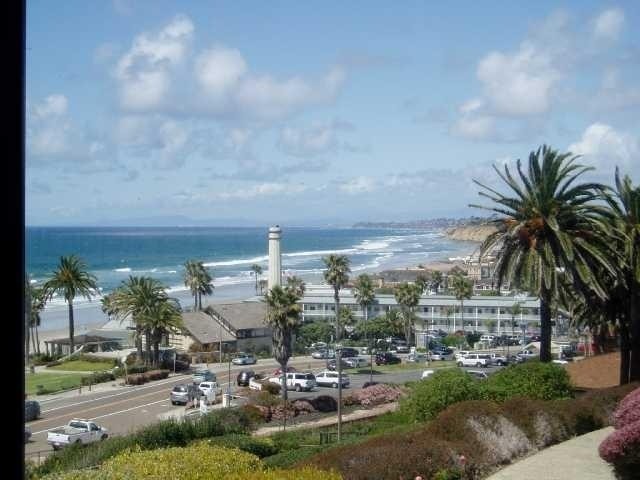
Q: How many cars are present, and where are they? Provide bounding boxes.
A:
[311,342,430,371]
[169,369,221,405]
[479,334,540,348]
[237,366,351,392]
[231,353,257,365]
[455,350,538,380]
[25,400,40,421]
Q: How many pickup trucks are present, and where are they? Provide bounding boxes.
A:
[47,417,111,451]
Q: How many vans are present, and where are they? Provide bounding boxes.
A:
[431,350,448,361]
